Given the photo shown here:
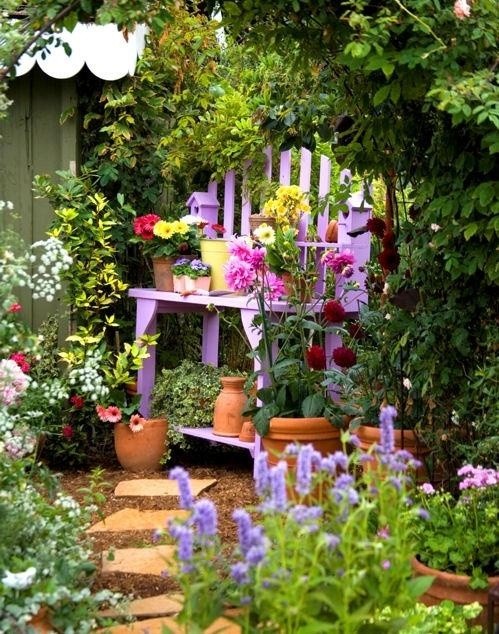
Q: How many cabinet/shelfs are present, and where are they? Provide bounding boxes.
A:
[128,143,372,481]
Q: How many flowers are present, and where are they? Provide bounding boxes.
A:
[222,232,358,436]
[415,465,499,591]
[133,181,311,280]
[307,206,430,429]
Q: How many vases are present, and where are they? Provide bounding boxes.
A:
[114,418,168,474]
[26,604,54,634]
[259,416,350,505]
[214,376,251,436]
[410,552,499,634]
[149,217,312,304]
[354,414,467,493]
